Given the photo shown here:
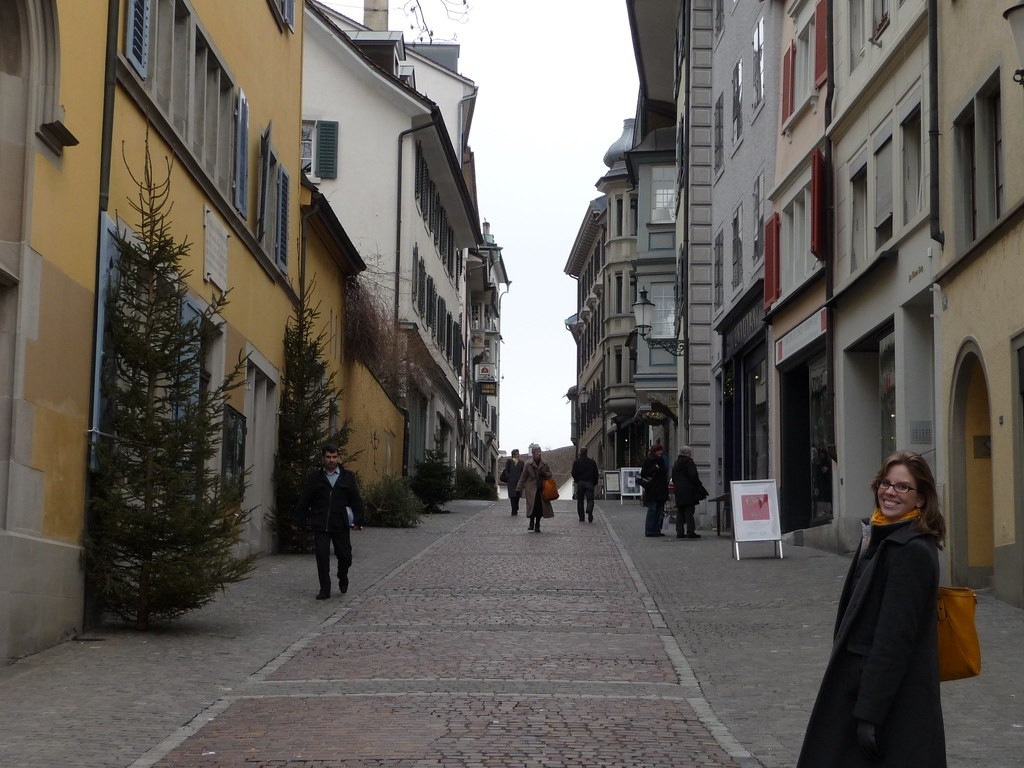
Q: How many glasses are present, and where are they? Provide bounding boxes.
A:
[876,479,918,493]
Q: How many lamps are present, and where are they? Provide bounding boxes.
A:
[632,286,685,357]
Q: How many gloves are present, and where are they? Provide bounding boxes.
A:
[853,719,878,758]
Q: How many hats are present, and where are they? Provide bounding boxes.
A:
[651,444,663,453]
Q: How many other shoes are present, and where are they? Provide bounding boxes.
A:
[337,572,348,593]
[316,591,330,600]
[687,533,701,538]
[586,509,593,522]
[579,518,585,521]
[676,533,687,537]
[528,526,534,530]
[534,527,540,532]
[645,532,665,537]
[512,507,519,516]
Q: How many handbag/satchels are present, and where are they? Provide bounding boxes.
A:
[542,474,559,501]
[500,459,510,483]
[634,475,652,488]
[935,587,982,682]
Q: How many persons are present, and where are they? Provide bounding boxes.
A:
[570,447,599,522]
[292,445,366,600]
[641,444,670,537]
[795,452,947,768]
[503,449,524,516]
[516,445,552,533]
[671,446,709,538]
